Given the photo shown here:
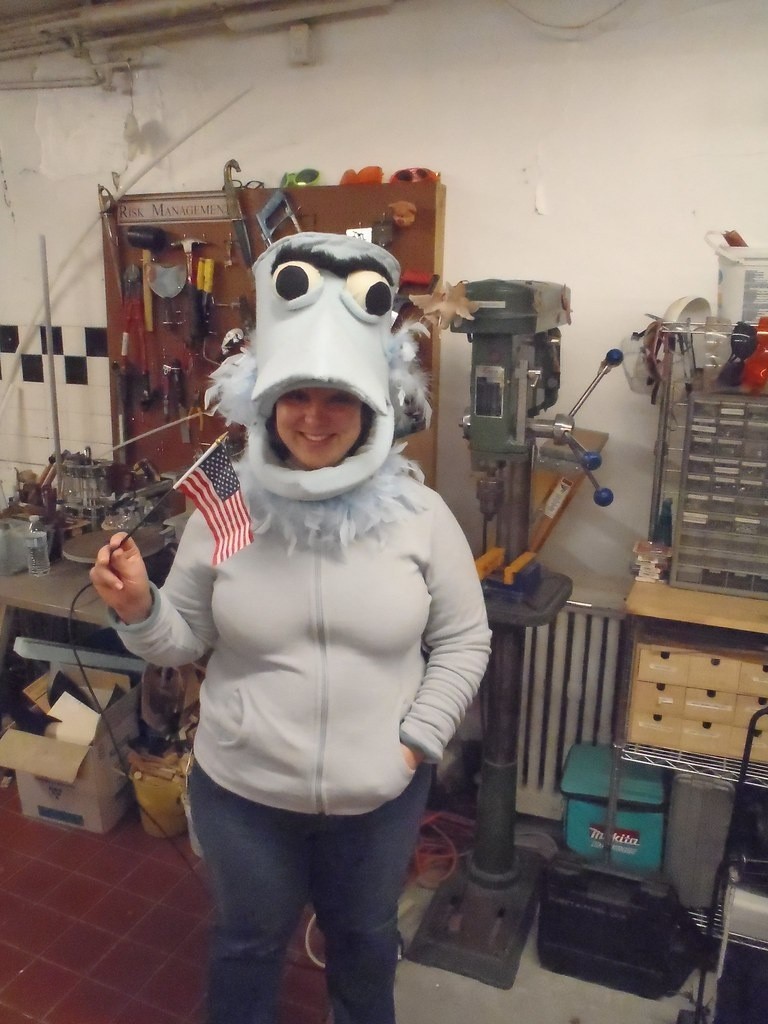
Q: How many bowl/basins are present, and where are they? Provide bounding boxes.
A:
[0,518,55,575]
[653,294,712,382]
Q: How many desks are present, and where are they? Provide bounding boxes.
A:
[0,561,113,663]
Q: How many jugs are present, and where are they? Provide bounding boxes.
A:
[60,445,114,519]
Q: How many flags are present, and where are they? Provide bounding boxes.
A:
[176,444,253,567]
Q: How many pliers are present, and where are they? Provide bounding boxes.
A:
[187,390,204,431]
[196,257,214,322]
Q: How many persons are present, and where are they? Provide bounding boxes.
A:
[92,233,493,1024]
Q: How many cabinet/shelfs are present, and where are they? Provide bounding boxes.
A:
[603,577,768,953]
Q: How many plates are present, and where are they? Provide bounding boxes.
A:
[62,530,164,563]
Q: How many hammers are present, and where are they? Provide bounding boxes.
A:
[128,226,165,332]
[170,238,207,339]
[137,328,157,413]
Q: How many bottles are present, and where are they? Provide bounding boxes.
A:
[26,515,50,577]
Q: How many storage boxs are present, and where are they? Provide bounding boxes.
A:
[560,744,667,877]
[629,629,767,764]
[1,664,143,834]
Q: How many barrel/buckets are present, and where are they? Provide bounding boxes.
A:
[703,228,767,325]
[127,751,195,839]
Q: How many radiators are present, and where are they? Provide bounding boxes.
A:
[514,586,625,819]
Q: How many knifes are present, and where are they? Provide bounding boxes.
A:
[223,160,254,270]
